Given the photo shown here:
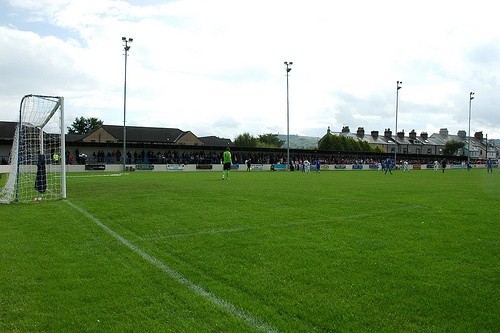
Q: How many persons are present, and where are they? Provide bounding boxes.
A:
[382,156,393,174]
[434,159,439,172]
[442,160,447,173]
[126,150,224,164]
[230,152,381,165]
[247,159,251,172]
[496,158,500,169]
[289,160,310,174]
[53,152,59,165]
[487,158,492,174]
[381,155,465,167]
[222,147,231,180]
[67,152,73,164]
[403,159,410,173]
[92,149,121,164]
[316,158,321,173]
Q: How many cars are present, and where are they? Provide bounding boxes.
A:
[78,153,87,158]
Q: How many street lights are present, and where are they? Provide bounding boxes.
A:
[467,92,474,168]
[284,61,293,172]
[121,36,133,173]
[394,81,402,170]
